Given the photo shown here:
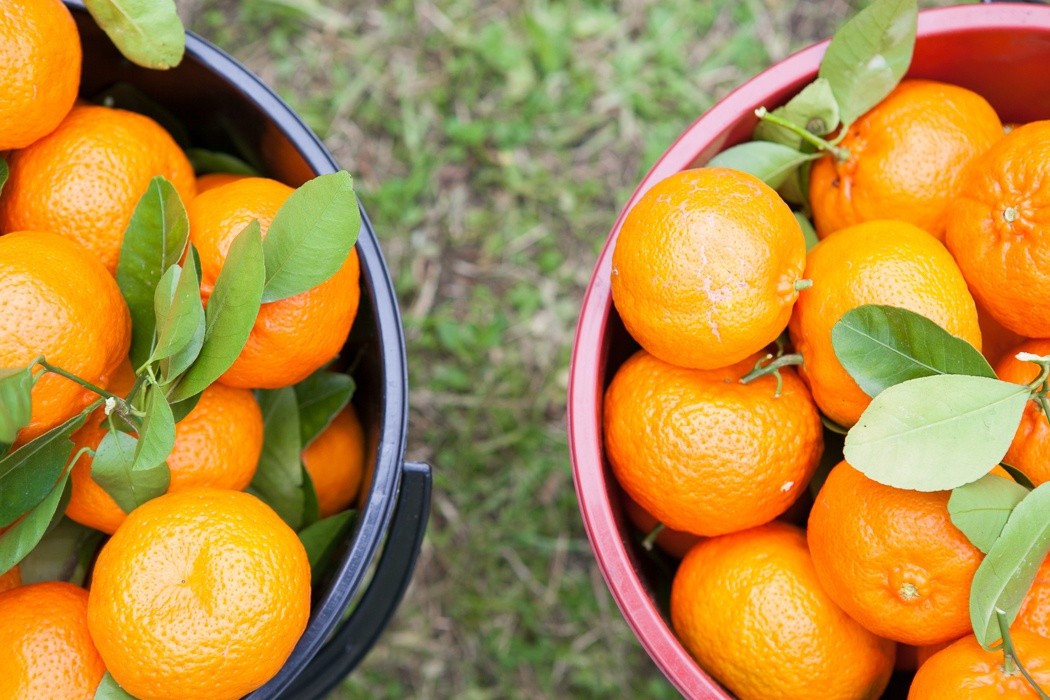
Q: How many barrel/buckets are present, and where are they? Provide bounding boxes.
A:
[62,0,432,700]
[568,2,1050,700]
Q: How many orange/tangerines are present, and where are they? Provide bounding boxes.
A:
[0,0,365,700]
[602,81,1050,700]
